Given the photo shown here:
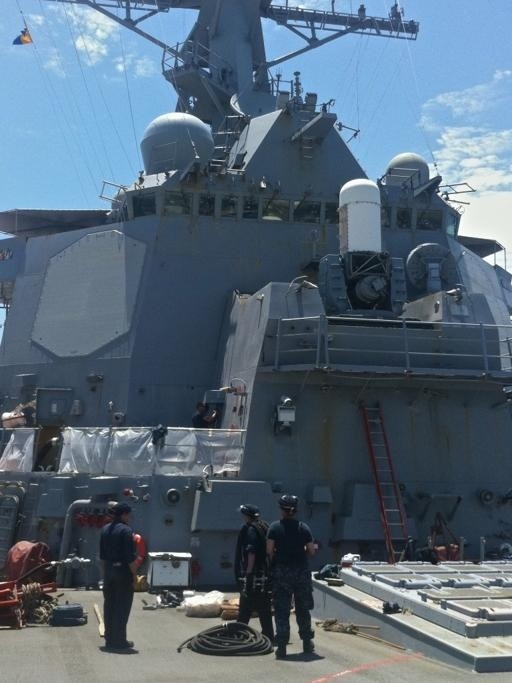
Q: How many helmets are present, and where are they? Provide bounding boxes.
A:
[278,494,299,512]
[239,504,261,518]
[196,401,208,410]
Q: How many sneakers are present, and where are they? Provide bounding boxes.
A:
[104,640,134,649]
[302,639,314,653]
[272,636,289,656]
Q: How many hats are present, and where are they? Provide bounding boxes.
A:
[109,502,132,515]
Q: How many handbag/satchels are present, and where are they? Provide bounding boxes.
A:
[236,572,270,594]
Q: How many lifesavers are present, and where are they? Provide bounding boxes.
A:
[132,533,145,567]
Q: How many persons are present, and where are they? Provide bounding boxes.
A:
[264,492,316,659]
[235,503,277,642]
[98,502,137,649]
[191,401,220,467]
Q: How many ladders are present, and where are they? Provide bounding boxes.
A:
[359,398,415,564]
